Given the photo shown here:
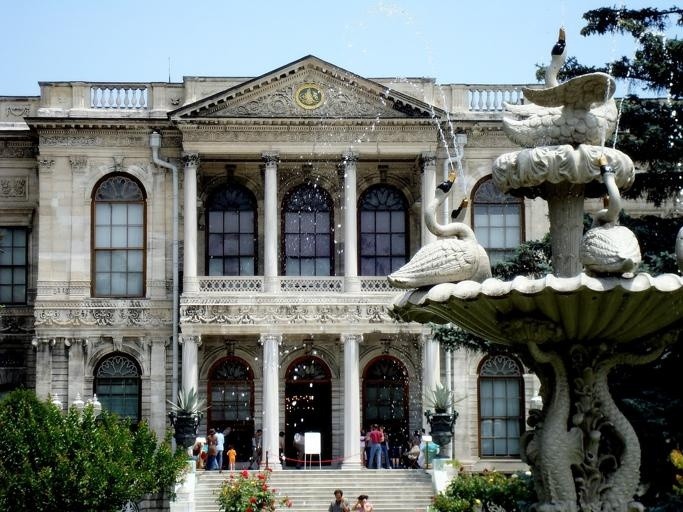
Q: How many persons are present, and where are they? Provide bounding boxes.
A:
[327,489,350,512]
[207,428,218,469]
[255,429,262,460]
[216,427,224,469]
[360,422,425,468]
[226,444,237,470]
[279,432,285,466]
[351,494,372,512]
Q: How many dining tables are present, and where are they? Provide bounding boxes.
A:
[420,381,469,458]
[166,387,211,460]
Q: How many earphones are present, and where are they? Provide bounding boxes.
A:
[451,197,494,283]
[388,172,477,288]
[500,27,619,146]
[578,155,646,277]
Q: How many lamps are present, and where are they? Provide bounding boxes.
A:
[403,450,420,468]
